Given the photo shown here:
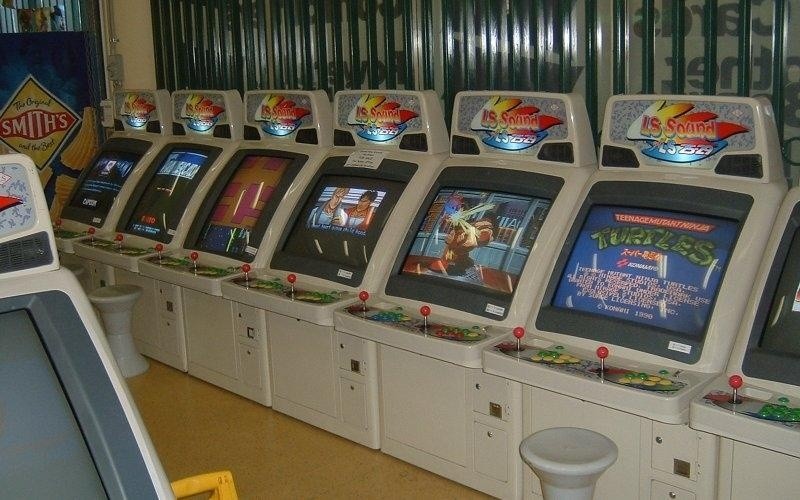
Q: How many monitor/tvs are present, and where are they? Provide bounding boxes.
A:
[741,201,800,385]
[535,181,754,365]
[385,166,565,321]
[182,148,310,263]
[116,142,223,244]
[270,155,419,288]
[0,289,158,499]
[60,138,153,229]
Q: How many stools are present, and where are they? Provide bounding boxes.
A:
[520,426,618,500]
[90,283,150,378]
